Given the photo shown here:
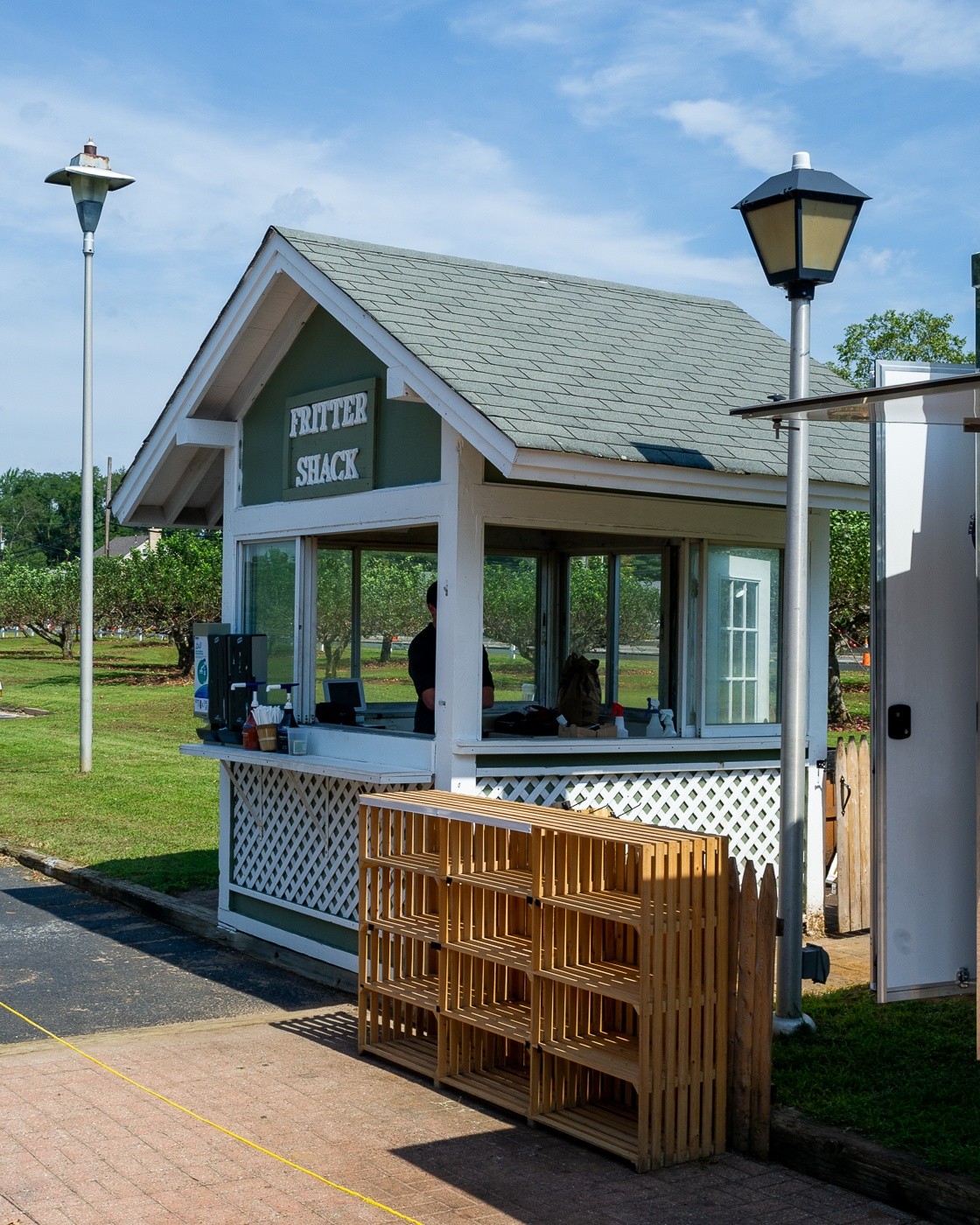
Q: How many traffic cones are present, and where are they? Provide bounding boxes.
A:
[860,637,871,668]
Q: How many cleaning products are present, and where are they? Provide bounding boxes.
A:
[660,708,676,736]
[266,682,301,754]
[645,698,665,736]
[610,702,629,738]
[230,680,267,749]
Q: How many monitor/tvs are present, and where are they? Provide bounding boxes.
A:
[322,679,366,712]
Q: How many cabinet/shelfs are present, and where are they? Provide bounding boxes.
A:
[358,789,728,1170]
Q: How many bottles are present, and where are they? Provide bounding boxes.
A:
[242,691,262,751]
[689,707,696,725]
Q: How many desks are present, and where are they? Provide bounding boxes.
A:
[179,743,432,856]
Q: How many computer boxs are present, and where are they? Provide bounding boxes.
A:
[354,716,365,723]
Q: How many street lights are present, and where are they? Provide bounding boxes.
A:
[731,150,872,1039]
[45,135,136,774]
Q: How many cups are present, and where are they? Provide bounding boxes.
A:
[287,727,310,756]
[255,724,278,752]
[522,684,536,701]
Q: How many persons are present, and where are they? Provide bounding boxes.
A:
[408,580,495,737]
